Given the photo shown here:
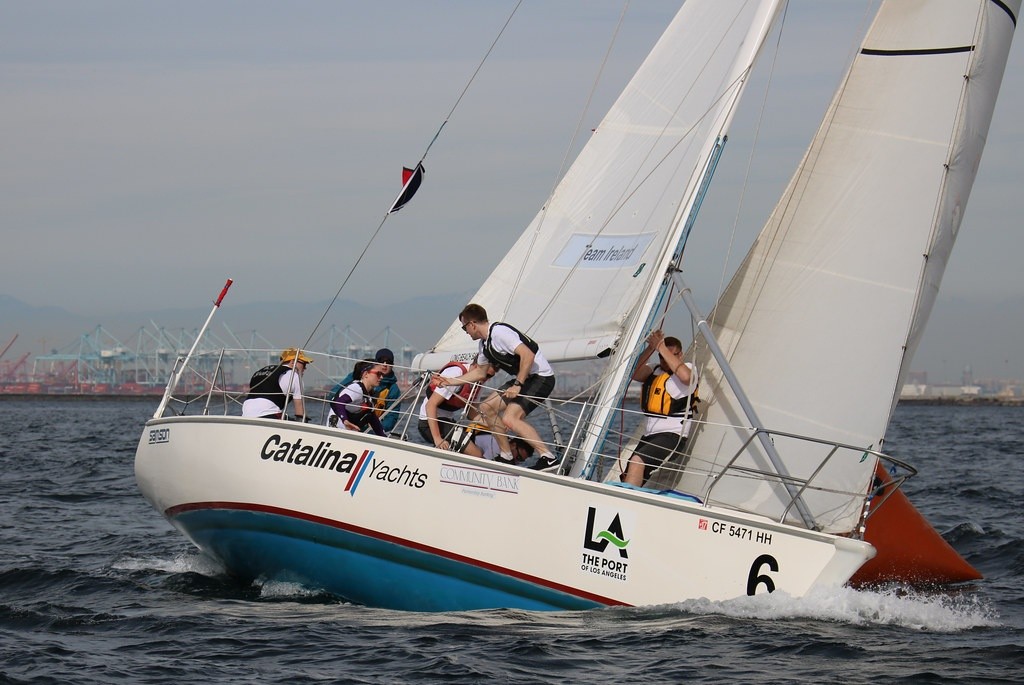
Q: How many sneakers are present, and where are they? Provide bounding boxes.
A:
[491,453,515,465]
[527,455,560,472]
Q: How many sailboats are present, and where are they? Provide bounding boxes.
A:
[134,0,1021,615]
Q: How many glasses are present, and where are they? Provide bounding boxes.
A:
[362,371,384,379]
[462,321,477,331]
[293,359,306,369]
[378,358,391,364]
[516,448,525,462]
[658,352,679,359]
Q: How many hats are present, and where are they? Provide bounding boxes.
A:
[280,347,313,363]
[376,348,393,364]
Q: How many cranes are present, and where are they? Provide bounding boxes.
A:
[0,317,422,386]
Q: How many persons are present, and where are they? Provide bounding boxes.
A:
[326,348,401,435]
[325,358,387,437]
[620,330,699,488]
[242,347,315,422]
[431,304,560,471]
[418,352,501,458]
[464,424,534,467]
